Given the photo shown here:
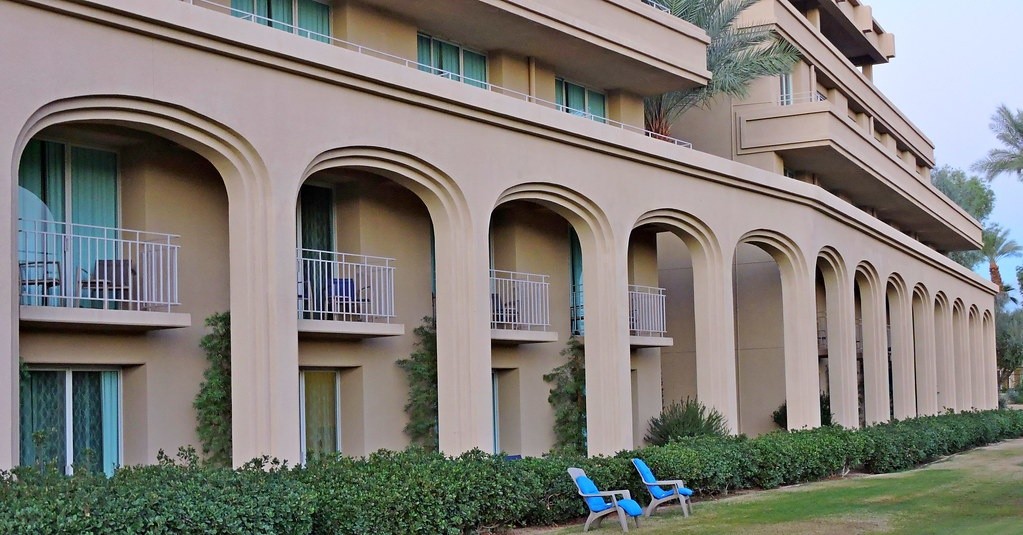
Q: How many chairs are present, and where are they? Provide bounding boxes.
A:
[631,458,694,519]
[490,293,520,330]
[327,278,371,322]
[567,468,643,534]
[76,260,137,310]
[19,261,61,306]
